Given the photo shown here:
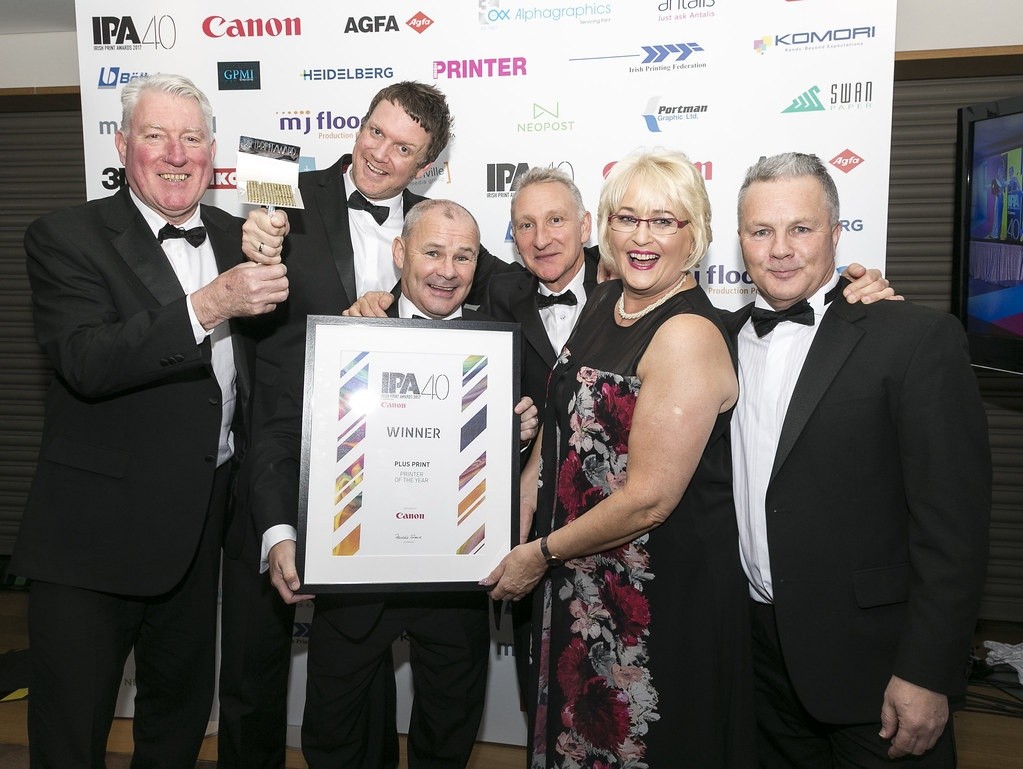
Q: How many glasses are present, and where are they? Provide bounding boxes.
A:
[608,214,691,236]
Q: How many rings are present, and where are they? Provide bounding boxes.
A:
[534,415,539,426]
[259,243,264,252]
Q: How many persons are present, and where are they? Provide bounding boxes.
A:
[219,82,620,769]
[7,74,290,769]
[480,146,749,769]
[725,153,991,769]
[248,202,539,769]
[341,169,905,769]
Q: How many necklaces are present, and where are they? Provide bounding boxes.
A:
[618,275,687,319]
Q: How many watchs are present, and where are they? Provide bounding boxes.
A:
[541,536,564,567]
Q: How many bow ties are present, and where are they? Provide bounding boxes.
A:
[346,190,390,226]
[751,275,842,336]
[156,222,206,248]
[412,314,464,322]
[537,290,579,310]
